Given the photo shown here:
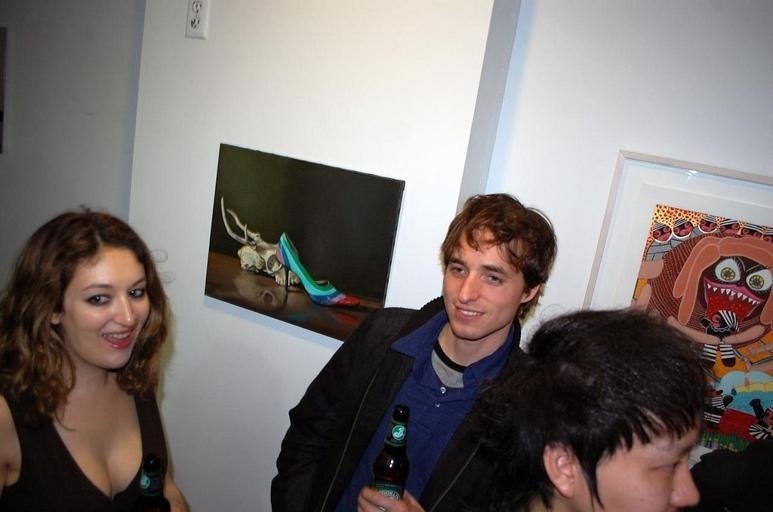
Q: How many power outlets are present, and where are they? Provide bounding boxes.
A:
[185,0,209,41]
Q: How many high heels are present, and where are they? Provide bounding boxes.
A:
[276,232,361,309]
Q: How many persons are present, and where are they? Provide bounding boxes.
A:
[477,308,709,511]
[269,196,556,511]
[0,207,189,511]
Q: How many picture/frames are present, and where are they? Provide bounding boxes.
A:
[581,149,773,465]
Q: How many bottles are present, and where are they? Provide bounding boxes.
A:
[131,456,171,512]
[368,404,411,511]
